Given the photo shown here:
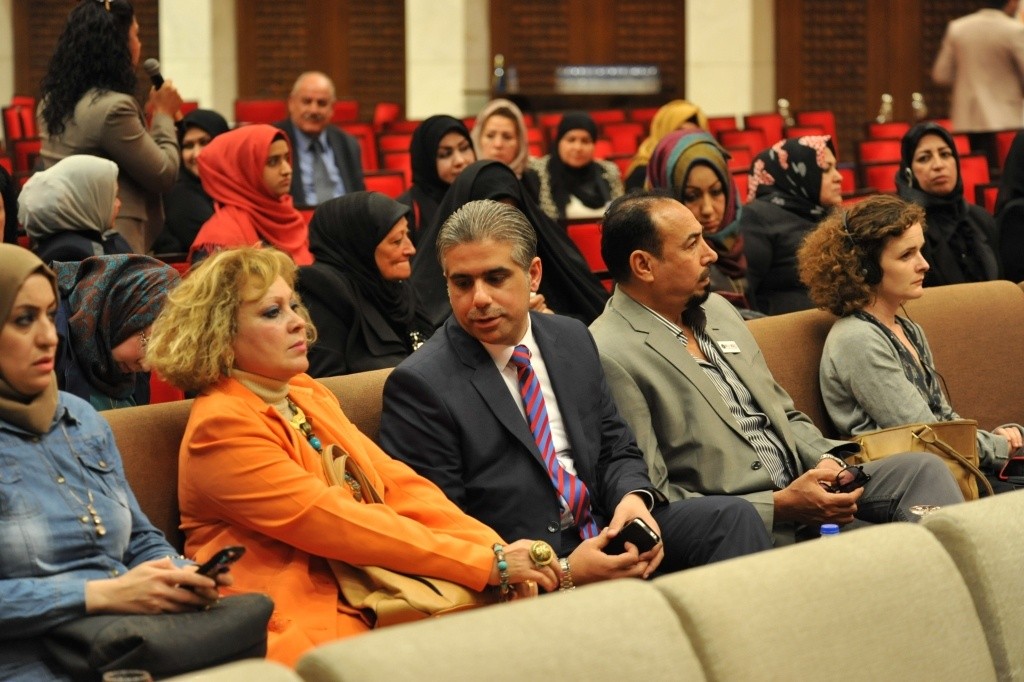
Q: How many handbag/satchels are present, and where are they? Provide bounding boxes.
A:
[316,444,493,629]
[37,593,277,681]
[844,420,996,503]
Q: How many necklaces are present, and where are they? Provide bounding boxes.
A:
[277,394,364,499]
[26,424,107,540]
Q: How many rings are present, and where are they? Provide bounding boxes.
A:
[525,540,554,571]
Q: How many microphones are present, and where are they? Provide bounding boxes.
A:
[143,58,184,124]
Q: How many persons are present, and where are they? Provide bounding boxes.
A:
[571,194,971,556]
[377,201,778,589]
[139,248,565,679]
[0,240,236,682]
[0,2,1024,410]
[791,195,1024,500]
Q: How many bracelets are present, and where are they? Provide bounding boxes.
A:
[814,453,848,470]
[553,551,577,594]
[492,543,512,598]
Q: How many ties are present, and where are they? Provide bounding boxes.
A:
[308,140,334,207]
[512,346,600,541]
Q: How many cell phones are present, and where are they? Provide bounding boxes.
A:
[602,518,660,555]
[180,545,246,592]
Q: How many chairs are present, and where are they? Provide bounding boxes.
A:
[661,524,999,682]
[294,578,708,682]
[920,480,1022,682]
[0,79,1024,551]
